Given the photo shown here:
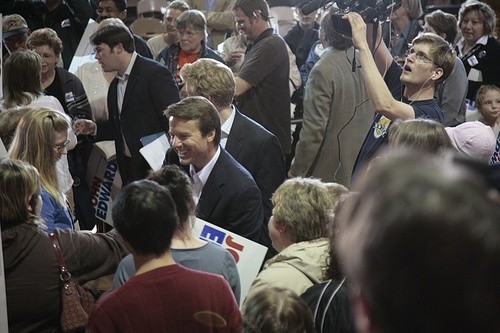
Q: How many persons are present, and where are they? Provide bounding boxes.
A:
[0,0,500,333]
[162,96,263,244]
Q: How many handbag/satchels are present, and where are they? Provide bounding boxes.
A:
[50,232,97,333]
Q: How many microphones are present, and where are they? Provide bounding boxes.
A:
[301,0,330,15]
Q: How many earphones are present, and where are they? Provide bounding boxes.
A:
[433,73,436,77]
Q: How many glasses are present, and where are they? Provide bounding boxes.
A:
[406,49,441,68]
[177,31,204,37]
[51,139,71,151]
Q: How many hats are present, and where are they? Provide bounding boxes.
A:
[444,121,497,164]
[2,14,28,39]
